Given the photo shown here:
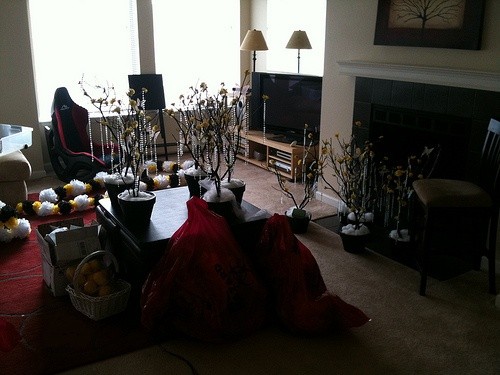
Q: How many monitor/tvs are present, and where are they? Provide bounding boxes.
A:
[250,72,321,147]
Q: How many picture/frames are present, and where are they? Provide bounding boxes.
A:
[373,0,487,51]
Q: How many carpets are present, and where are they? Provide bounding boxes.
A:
[0,185,175,375]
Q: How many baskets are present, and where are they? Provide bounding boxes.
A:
[65,249,132,322]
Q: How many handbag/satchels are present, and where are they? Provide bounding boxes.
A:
[245,214,371,336]
[139,196,267,348]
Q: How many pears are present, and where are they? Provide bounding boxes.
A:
[64,261,114,297]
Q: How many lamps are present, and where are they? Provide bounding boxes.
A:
[240,29,269,70]
[285,29,314,74]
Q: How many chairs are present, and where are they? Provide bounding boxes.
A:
[43,87,124,183]
[405,117,500,298]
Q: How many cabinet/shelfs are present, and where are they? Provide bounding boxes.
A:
[227,124,319,182]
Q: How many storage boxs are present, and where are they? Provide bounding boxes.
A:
[0,124,34,158]
[35,216,101,298]
[44,224,100,262]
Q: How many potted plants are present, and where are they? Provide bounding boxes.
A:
[78,73,442,261]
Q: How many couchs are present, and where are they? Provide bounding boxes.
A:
[0,150,32,205]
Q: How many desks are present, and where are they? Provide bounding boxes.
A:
[95,183,280,342]
